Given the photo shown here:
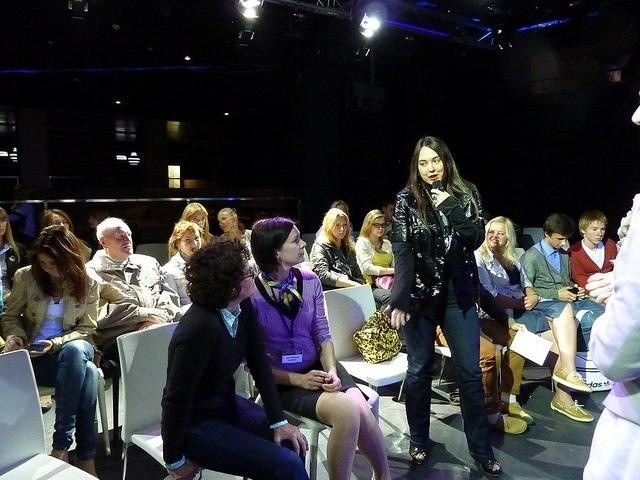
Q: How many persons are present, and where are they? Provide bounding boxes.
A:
[83,217,180,361]
[433,281,535,435]
[2,224,100,480]
[582,86,640,480]
[158,193,397,311]
[566,210,618,297]
[384,135,502,477]
[0,206,96,354]
[518,211,606,352]
[472,215,594,423]
[162,238,310,480]
[238,216,394,480]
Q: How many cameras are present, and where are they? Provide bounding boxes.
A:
[568,287,579,295]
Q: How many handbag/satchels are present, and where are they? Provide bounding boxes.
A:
[353,311,402,363]
[374,275,394,291]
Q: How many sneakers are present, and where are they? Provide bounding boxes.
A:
[37,394,53,411]
[550,397,594,422]
[481,400,533,435]
[51,449,70,463]
[552,367,593,393]
[77,460,97,478]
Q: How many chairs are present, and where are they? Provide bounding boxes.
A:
[248,383,380,480]
[300,230,360,256]
[524,228,571,253]
[116,321,207,480]
[136,244,172,266]
[0,348,98,480]
[322,283,408,402]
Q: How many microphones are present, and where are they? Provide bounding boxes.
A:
[432,180,452,232]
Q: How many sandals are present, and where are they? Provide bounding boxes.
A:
[474,456,501,476]
[409,441,429,463]
